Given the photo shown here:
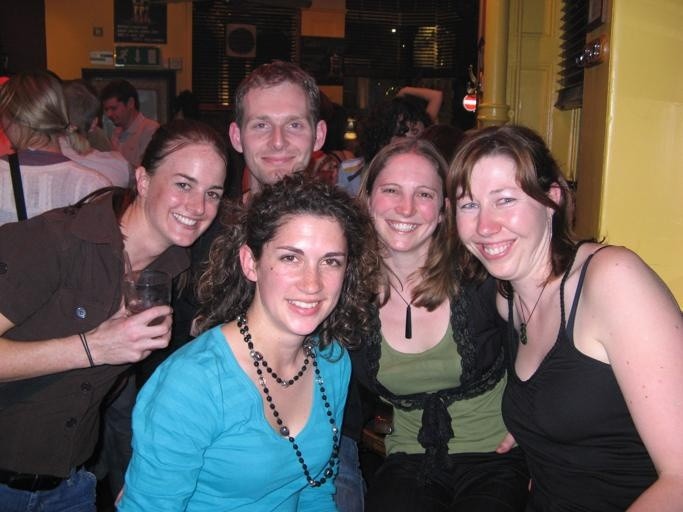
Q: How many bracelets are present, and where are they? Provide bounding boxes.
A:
[78,329,97,370]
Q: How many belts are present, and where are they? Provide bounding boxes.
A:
[0,470,65,493]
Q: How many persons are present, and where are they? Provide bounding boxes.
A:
[0,64,140,512]
[341,132,533,512]
[129,54,343,395]
[110,161,378,512]
[441,117,682,510]
[0,109,241,510]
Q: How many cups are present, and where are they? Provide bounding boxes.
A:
[120,270,173,331]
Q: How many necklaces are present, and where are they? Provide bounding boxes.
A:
[235,310,340,487]
[387,279,424,342]
[242,316,312,391]
[515,277,551,344]
